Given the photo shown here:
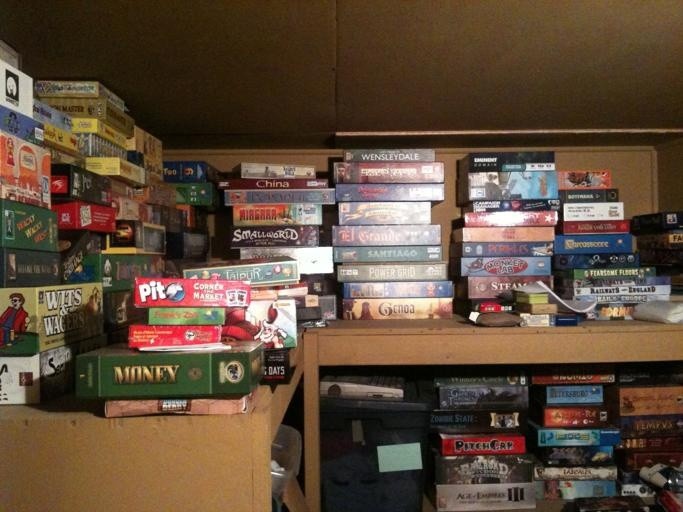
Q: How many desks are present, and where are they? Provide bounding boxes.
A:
[2,334,302,511]
[299,317,683,510]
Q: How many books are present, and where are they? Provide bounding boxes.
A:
[510,276,597,315]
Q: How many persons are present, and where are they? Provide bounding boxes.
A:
[0,290,32,350]
